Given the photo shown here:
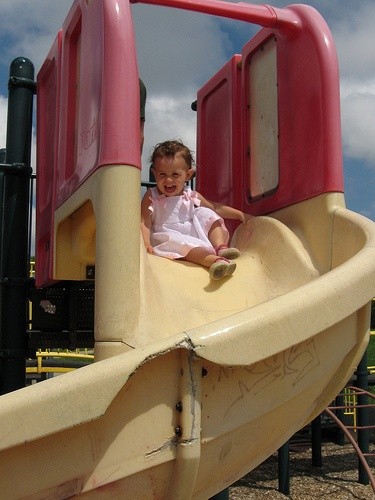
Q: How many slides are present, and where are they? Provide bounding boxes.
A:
[0,166,375,500]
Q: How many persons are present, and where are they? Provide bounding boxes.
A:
[141,141,255,280]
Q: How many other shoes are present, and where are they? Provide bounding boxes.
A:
[216,243,240,259]
[209,257,237,281]
